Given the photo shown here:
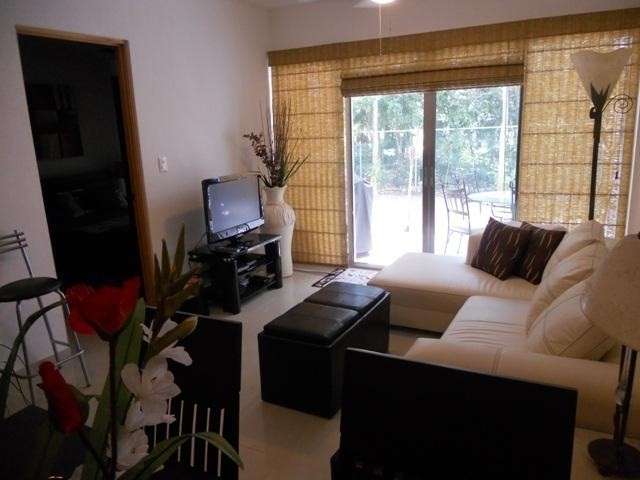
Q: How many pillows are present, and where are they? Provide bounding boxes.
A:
[526,278,618,362]
[523,242,608,338]
[540,220,606,284]
[513,218,566,286]
[470,216,532,282]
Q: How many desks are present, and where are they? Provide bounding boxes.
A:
[466,190,516,225]
[1,403,91,479]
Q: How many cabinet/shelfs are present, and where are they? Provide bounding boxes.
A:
[187,233,283,315]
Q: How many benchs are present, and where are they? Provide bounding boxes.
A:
[255,281,393,420]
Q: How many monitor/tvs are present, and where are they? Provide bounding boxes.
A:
[201,171,265,249]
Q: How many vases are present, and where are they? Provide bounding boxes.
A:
[259,184,298,279]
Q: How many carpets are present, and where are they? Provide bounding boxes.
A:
[311,264,381,290]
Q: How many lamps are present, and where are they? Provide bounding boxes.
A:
[576,234,640,480]
[569,47,634,221]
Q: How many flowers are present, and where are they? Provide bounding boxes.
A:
[241,92,311,188]
[1,224,243,479]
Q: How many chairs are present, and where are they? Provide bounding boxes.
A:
[136,306,244,480]
[0,227,91,407]
[439,184,483,257]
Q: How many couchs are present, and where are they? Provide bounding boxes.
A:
[397,294,639,441]
[366,234,620,335]
